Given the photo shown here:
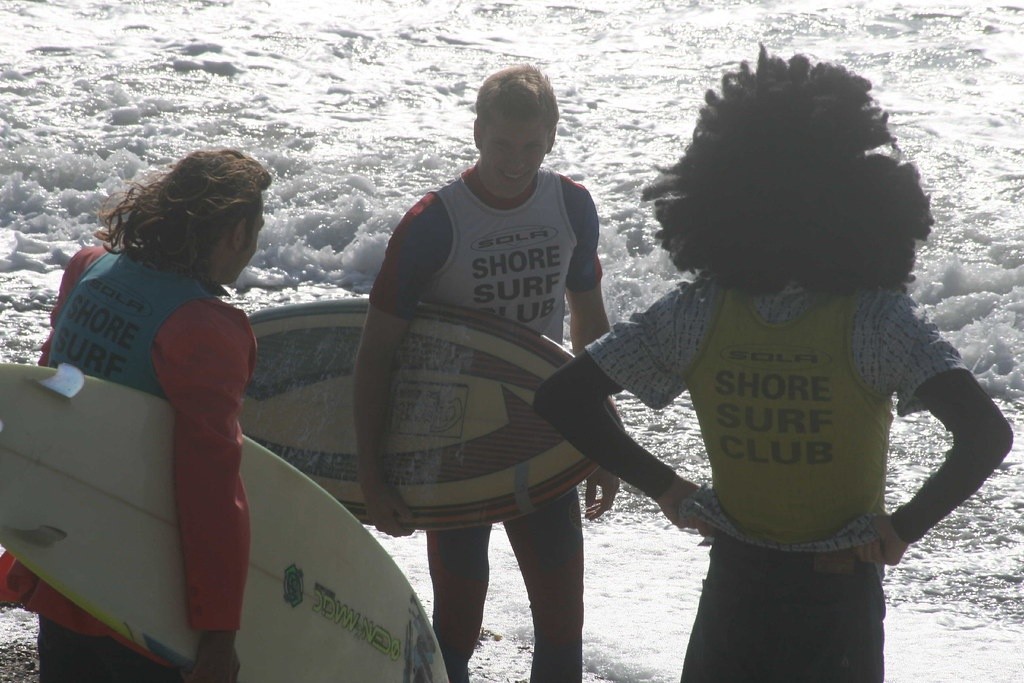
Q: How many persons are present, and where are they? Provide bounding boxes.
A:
[0,148,271,683]
[534,41,1014,683]
[355,64,620,683]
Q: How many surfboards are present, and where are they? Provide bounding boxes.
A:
[232,302,619,530]
[0,365,450,683]
